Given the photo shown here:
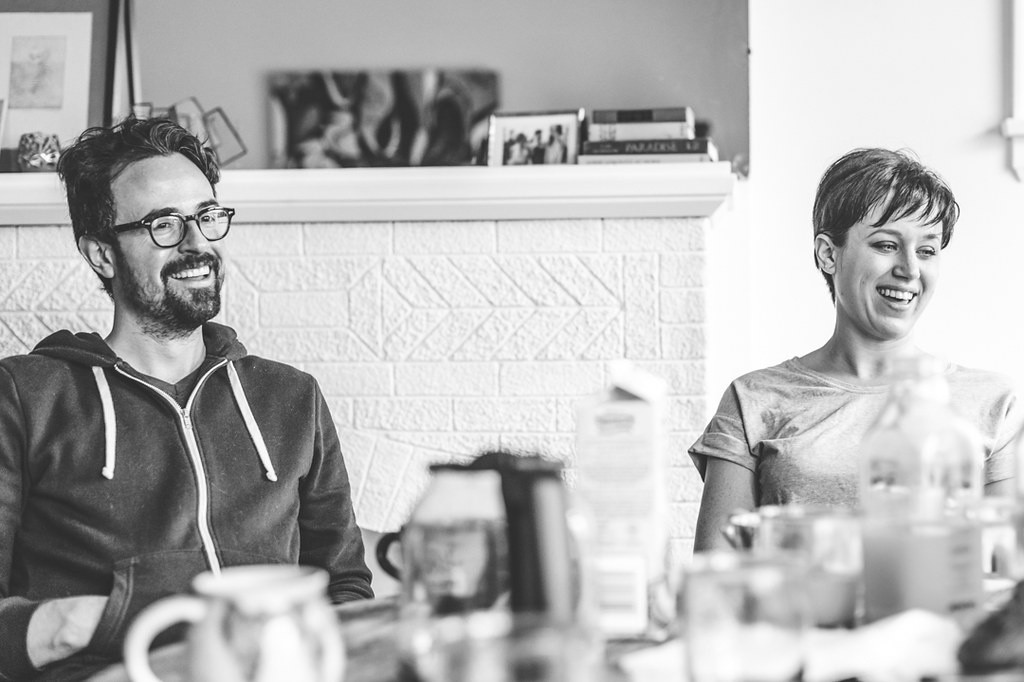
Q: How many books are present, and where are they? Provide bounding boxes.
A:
[576,107,720,164]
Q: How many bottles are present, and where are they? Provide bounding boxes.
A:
[855,356,984,635]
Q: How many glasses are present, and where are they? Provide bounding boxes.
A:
[112,206,235,249]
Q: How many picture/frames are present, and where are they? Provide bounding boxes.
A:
[0,0,120,173]
[485,106,585,165]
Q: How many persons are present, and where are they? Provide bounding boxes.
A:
[504,124,567,166]
[0,116,370,682]
[687,149,1024,579]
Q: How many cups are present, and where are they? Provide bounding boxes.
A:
[752,502,862,629]
[121,563,346,682]
[678,547,807,682]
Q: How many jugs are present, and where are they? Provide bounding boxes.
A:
[396,450,581,682]
